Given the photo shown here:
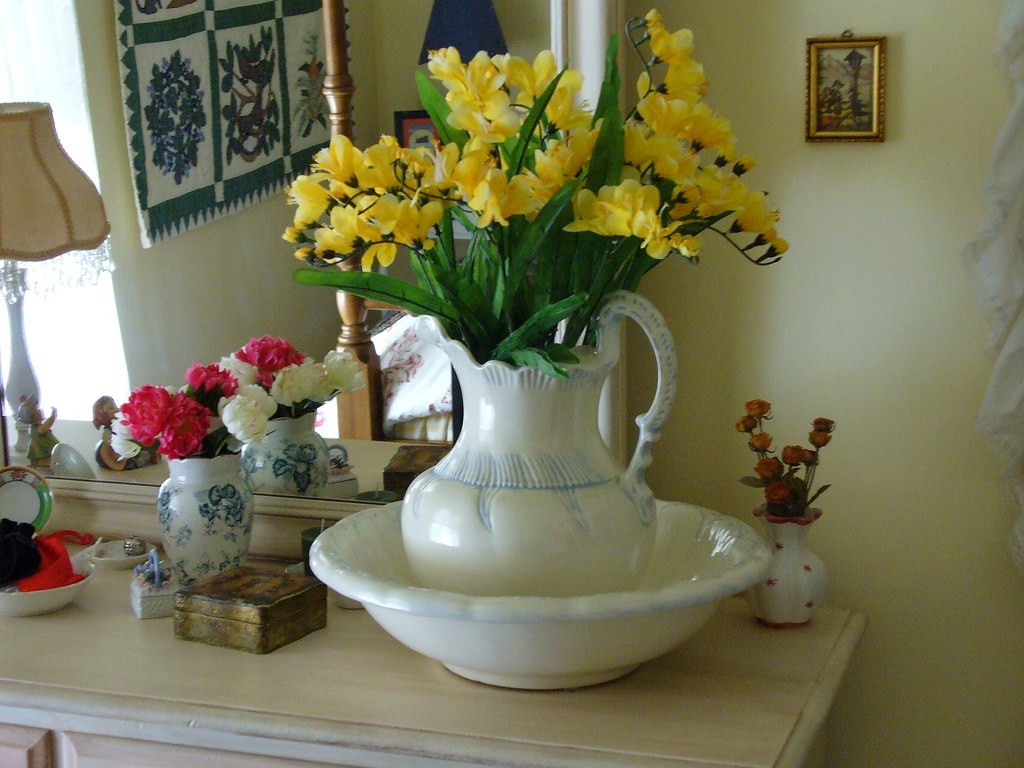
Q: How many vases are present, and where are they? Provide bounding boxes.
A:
[744,501,831,628]
[238,410,349,497]
[401,290,679,597]
[155,455,256,586]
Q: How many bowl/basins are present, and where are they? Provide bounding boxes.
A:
[308,500,772,690]
[0,556,98,616]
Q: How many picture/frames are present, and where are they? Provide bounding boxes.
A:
[395,106,442,149]
[0,0,627,517]
[804,27,891,143]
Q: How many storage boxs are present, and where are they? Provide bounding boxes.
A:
[175,564,327,654]
[383,445,455,500]
[328,472,359,498]
[130,551,181,620]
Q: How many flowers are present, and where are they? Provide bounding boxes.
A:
[119,360,276,464]
[282,3,789,383]
[221,335,370,416]
[736,399,834,516]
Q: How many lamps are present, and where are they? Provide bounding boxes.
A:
[0,101,114,449]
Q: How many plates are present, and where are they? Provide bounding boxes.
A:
[0,466,54,537]
[50,444,97,481]
[82,540,158,569]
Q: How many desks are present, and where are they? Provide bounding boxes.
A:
[0,544,869,767]
[5,418,441,499]
